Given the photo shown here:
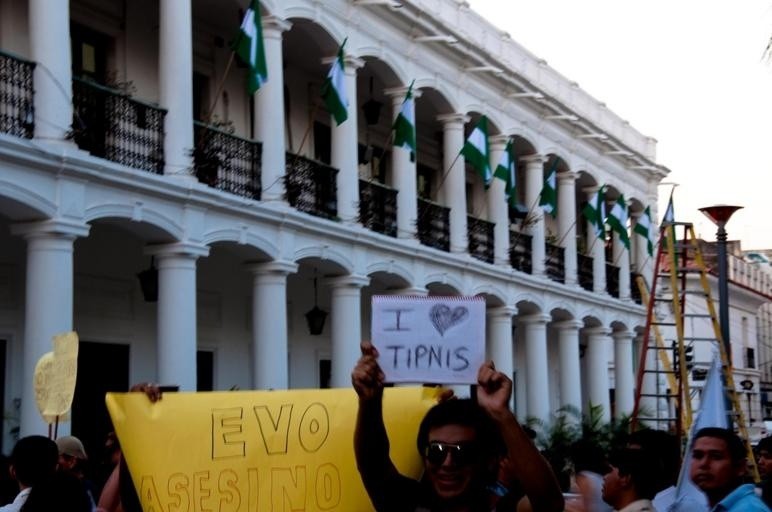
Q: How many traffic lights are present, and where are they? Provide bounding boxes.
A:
[684,344,694,372]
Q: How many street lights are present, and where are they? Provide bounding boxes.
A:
[697,203,745,430]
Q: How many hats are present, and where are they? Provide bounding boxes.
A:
[417,398,500,456]
[54,435,89,461]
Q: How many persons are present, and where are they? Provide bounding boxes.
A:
[602,449,659,512]
[689,427,772,512]
[624,428,676,512]
[1,381,162,512]
[755,437,772,509]
[351,342,565,512]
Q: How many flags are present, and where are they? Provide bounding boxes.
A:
[583,185,674,259]
[493,138,520,207]
[233,1,268,95]
[539,169,557,219]
[460,115,493,186]
[391,90,416,150]
[320,48,350,126]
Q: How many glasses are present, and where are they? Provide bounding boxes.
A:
[425,440,477,464]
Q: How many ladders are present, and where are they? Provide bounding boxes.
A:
[629,222,762,485]
